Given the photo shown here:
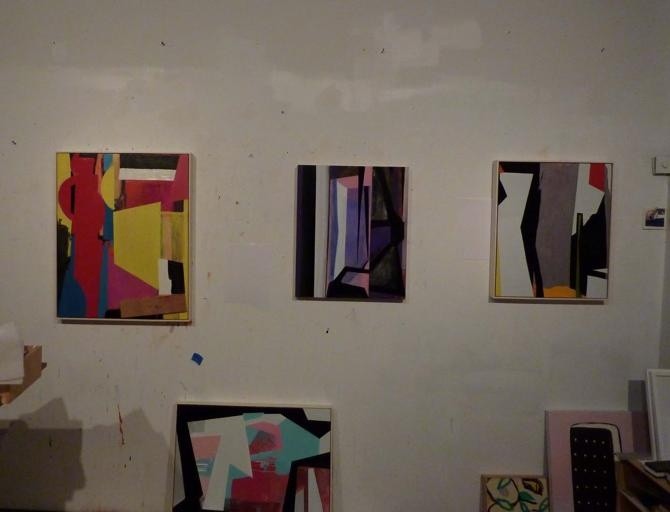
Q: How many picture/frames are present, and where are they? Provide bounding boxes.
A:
[643,366,670,466]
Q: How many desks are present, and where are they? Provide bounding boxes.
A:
[614,452,670,512]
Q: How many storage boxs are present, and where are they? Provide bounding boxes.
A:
[0,341,44,406]
